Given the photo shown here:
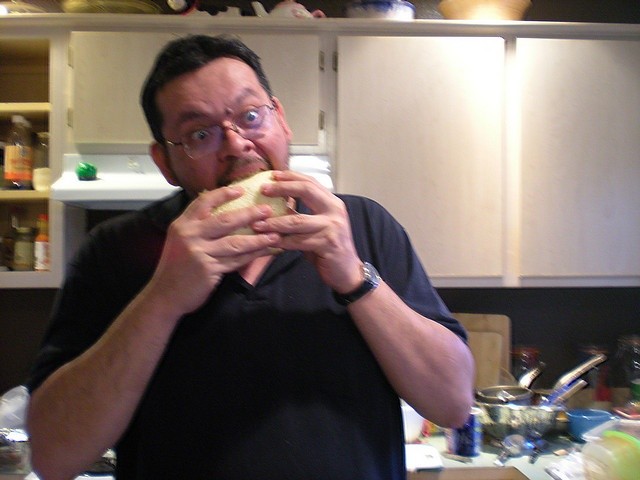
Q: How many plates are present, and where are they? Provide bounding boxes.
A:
[2,2,49,13]
[58,0,164,14]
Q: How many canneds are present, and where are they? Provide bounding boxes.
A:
[11,226,37,270]
[444,406,482,461]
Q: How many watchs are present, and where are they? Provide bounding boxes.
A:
[331,261,381,307]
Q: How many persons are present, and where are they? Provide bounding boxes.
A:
[23,30,476,480]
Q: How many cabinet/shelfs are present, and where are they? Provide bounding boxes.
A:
[0,25,67,288]
[336,36,640,288]
[69,31,322,148]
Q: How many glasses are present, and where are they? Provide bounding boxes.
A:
[164,106,277,160]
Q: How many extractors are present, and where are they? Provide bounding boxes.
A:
[51,153,336,211]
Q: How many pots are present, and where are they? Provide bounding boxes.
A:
[477,362,545,405]
[473,352,607,442]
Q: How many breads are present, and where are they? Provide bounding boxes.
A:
[196,169,296,256]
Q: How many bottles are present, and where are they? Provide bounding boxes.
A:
[35,214,49,270]
[13,227,41,271]
[32,132,48,190]
[4,115,32,189]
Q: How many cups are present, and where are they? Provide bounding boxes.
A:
[251,0,326,18]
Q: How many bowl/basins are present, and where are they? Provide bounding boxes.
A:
[565,408,612,442]
[345,0,415,19]
[436,0,534,21]
